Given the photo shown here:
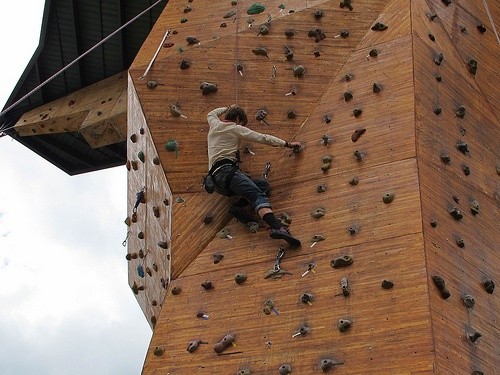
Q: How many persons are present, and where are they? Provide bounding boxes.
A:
[206,105,302,249]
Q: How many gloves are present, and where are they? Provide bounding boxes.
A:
[287,140,302,152]
[228,104,240,110]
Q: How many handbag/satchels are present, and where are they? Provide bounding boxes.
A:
[204,175,217,193]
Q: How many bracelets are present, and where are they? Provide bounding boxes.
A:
[285,141,288,147]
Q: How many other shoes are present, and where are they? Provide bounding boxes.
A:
[270,227,301,248]
[229,205,254,225]
